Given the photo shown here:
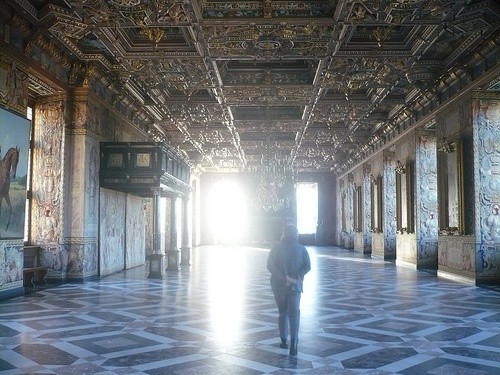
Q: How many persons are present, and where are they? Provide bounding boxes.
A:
[265,224,313,357]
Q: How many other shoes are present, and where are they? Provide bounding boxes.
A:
[280,338,289,349]
[290,345,298,355]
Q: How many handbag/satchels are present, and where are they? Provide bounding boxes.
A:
[284,276,303,292]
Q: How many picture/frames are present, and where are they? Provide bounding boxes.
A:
[0,105,33,242]
